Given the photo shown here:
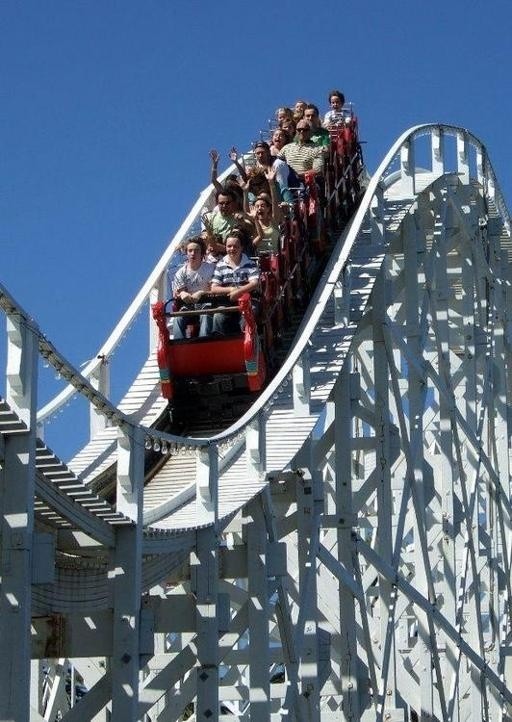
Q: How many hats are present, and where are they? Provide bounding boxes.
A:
[256,140,269,147]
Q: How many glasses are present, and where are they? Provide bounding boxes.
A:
[218,199,234,205]
[297,126,311,132]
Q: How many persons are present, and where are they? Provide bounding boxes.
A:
[275,106,290,121]
[201,189,254,260]
[235,166,282,294]
[169,236,212,339]
[252,141,305,232]
[209,230,260,336]
[268,128,289,158]
[323,90,352,132]
[301,104,332,156]
[293,100,305,120]
[276,119,324,186]
[208,145,248,208]
[279,118,296,136]
